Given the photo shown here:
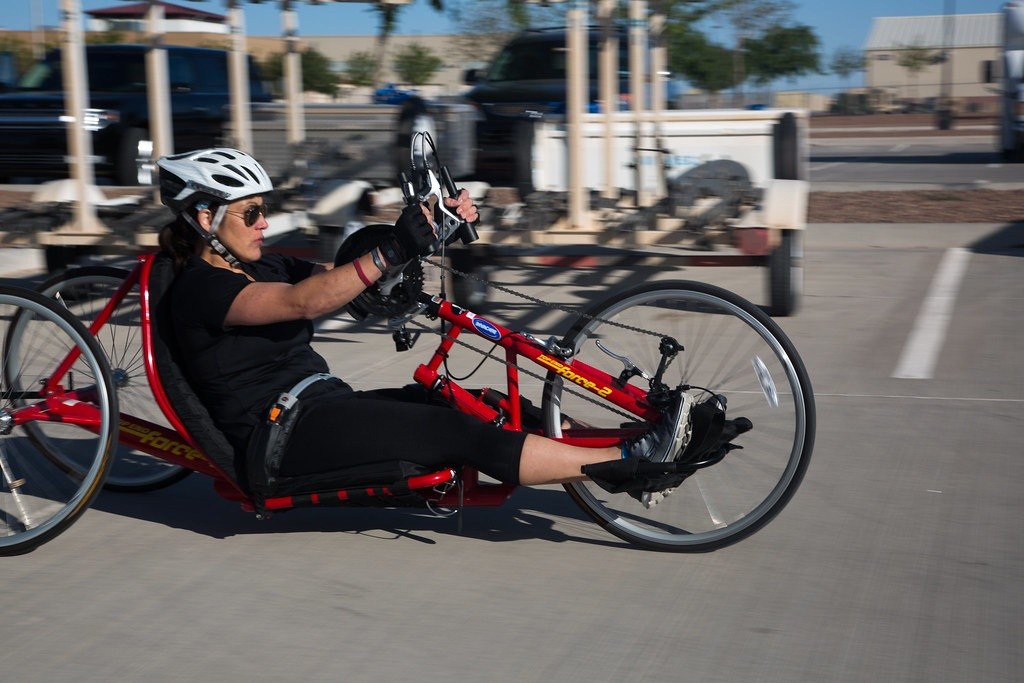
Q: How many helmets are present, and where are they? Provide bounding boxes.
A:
[156,148,274,214]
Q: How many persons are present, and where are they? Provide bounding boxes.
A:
[153,148,728,510]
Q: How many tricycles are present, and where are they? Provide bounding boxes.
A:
[0,130,817,557]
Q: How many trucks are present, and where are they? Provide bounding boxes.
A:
[976,0,1023,161]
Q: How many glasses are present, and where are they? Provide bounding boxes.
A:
[208,203,268,226]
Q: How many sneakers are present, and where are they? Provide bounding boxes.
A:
[623,393,694,508]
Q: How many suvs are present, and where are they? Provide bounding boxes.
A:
[0,41,276,188]
[459,15,681,186]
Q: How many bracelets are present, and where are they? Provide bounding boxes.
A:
[372,246,387,273]
[352,258,373,288]
